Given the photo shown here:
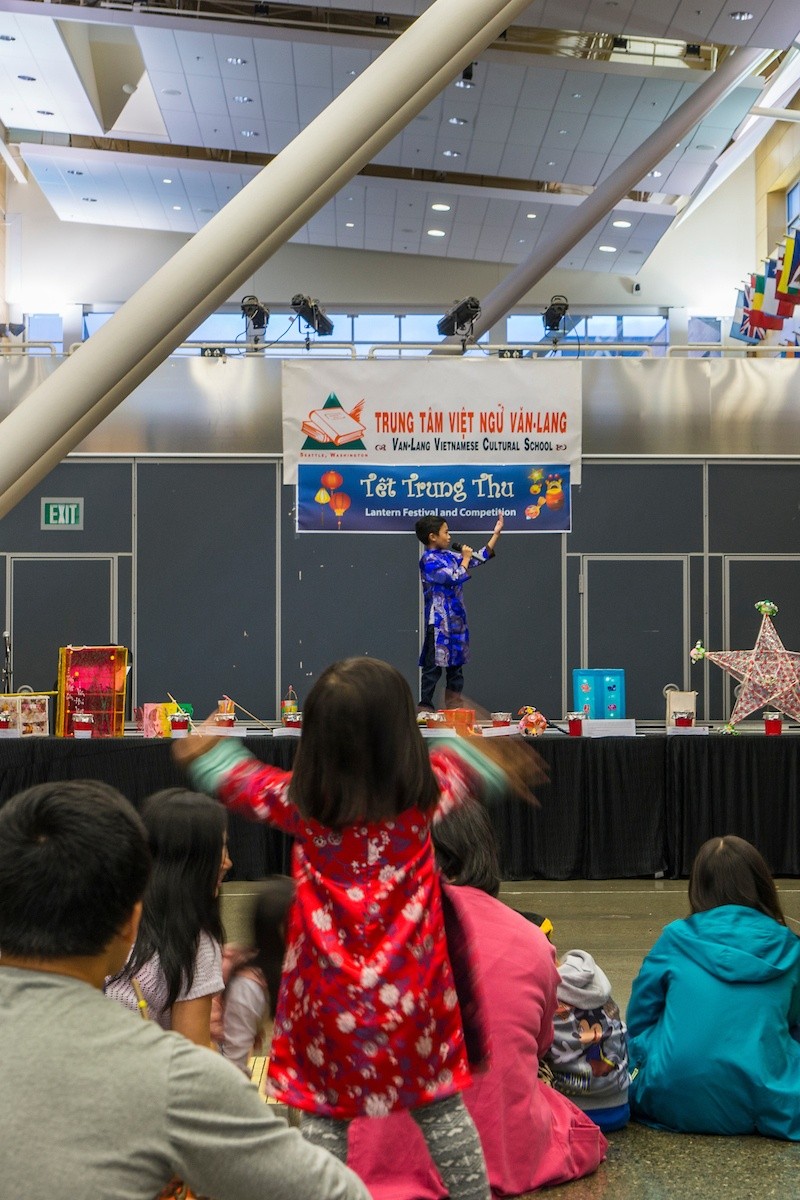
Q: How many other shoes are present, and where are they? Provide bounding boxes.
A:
[416,711,430,724]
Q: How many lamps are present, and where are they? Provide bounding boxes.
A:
[437,296,481,351]
[288,293,334,351]
[240,295,270,345]
[542,294,569,344]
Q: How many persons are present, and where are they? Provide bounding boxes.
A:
[415,511,503,725]
[0,653,639,1200]
[625,836,800,1140]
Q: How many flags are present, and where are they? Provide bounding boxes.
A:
[729,227,800,358]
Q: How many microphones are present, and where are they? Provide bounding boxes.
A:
[452,542,487,564]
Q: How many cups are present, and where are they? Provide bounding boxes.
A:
[133,702,194,738]
[567,713,583,736]
[282,699,298,727]
[72,714,94,739]
[674,711,694,727]
[213,700,237,727]
[490,712,512,728]
[284,712,304,728]
[428,708,475,735]
[763,712,783,736]
